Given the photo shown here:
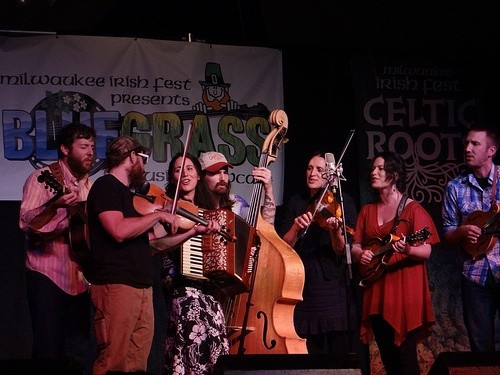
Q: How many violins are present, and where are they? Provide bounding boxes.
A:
[133,182,238,246]
[307,189,357,238]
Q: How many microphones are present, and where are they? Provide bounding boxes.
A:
[325,153,336,193]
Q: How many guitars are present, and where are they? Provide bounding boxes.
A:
[36,170,92,284]
[463,201,500,259]
[356,226,431,281]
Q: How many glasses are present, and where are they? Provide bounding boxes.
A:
[129,151,149,164]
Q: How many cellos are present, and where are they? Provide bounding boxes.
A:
[223,109,309,354]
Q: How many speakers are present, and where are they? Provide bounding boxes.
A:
[213,353,361,375]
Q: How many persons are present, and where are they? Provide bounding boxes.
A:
[274,150,368,374]
[83,135,221,375]
[18,123,107,375]
[198,151,276,226]
[350,151,440,374]
[148,151,230,375]
[441,124,499,366]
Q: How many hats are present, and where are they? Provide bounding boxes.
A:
[106,136,145,164]
[198,151,234,172]
